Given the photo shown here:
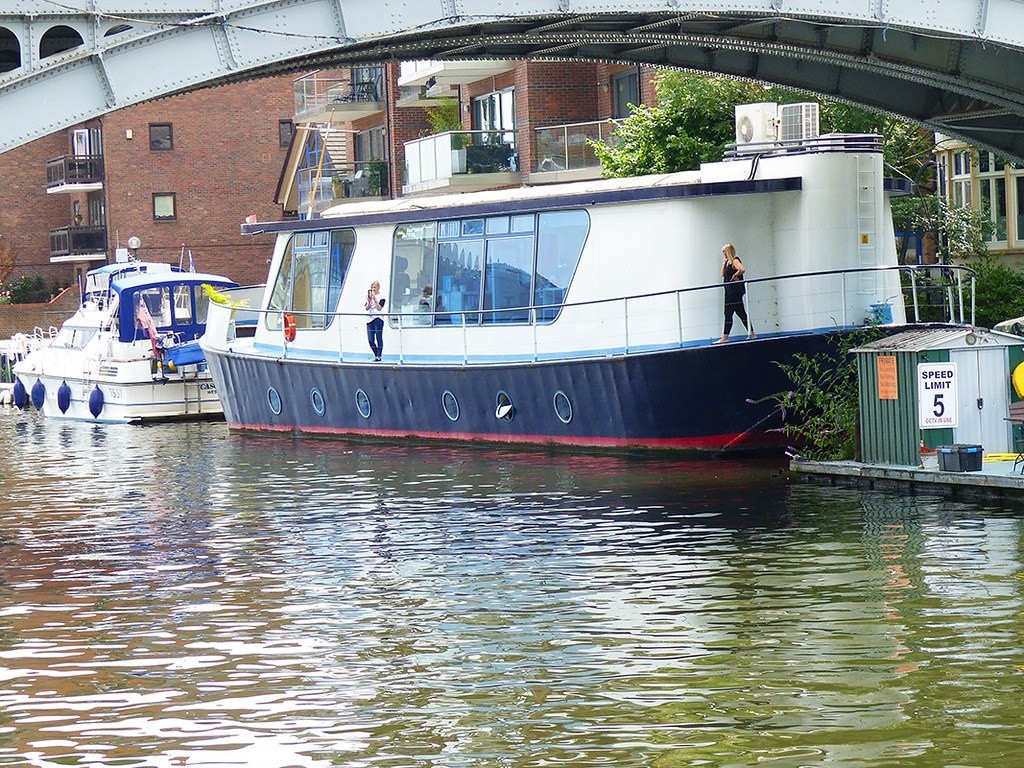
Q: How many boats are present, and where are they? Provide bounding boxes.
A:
[198,103,975,451]
[1,230,241,425]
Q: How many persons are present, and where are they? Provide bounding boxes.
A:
[712,242,757,344]
[418,286,433,325]
[74,215,88,226]
[363,281,385,362]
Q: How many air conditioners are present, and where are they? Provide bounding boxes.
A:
[734,103,820,156]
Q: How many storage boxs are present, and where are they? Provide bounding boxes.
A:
[935,443,984,472]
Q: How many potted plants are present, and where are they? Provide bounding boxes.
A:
[332,173,343,197]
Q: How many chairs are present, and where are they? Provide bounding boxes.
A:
[1009,400,1024,475]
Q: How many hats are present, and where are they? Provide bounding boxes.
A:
[168,331,174,335]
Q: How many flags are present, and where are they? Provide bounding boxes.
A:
[139,297,161,363]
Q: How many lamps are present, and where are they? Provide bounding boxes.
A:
[425,76,436,89]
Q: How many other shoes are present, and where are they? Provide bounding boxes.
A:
[747,336,757,339]
[372,358,381,362]
[712,339,729,344]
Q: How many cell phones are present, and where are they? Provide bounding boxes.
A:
[368,290,372,294]
